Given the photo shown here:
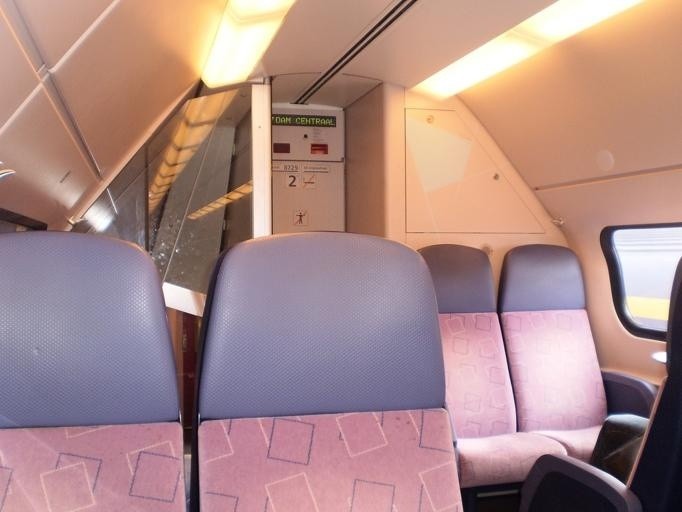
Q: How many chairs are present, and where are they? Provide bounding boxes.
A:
[515,255,682,512]
[497,241,659,464]
[1,229,190,512]
[192,231,466,511]
[416,241,569,500]
